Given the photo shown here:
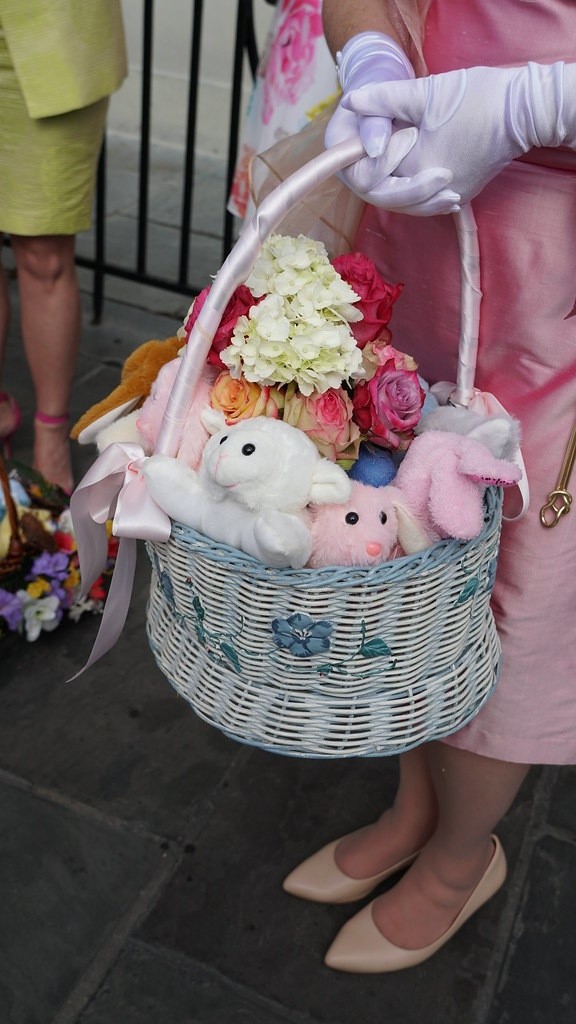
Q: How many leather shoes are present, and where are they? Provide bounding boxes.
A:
[283,815,425,904]
[324,834,508,973]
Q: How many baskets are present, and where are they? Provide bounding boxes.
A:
[144,132,501,759]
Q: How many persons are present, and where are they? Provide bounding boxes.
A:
[0,0,130,497]
[284,0,576,972]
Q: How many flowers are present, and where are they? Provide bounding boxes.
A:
[0,509,120,642]
[180,232,428,464]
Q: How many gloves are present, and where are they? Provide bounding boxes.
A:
[341,61,576,205]
[324,31,461,218]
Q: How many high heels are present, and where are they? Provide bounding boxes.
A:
[0,393,20,460]
[32,411,73,499]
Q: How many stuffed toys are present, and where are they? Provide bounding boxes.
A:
[69,336,521,568]
